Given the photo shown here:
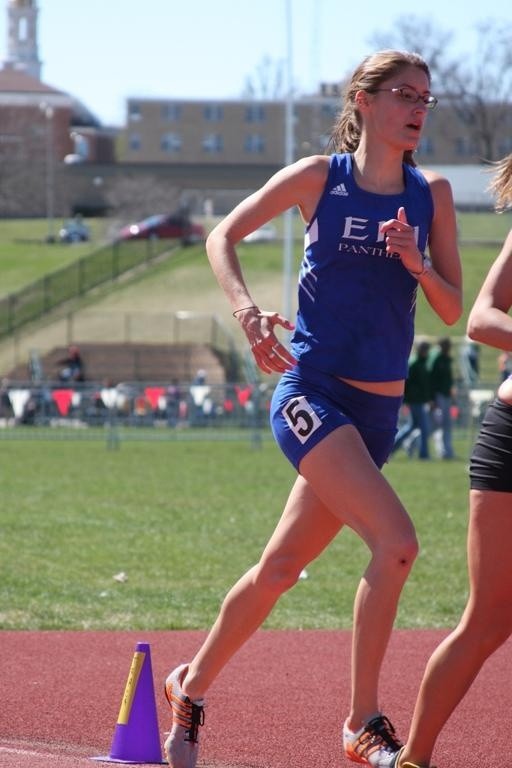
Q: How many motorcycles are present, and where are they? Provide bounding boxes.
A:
[43,225,89,243]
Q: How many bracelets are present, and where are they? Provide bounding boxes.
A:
[233,306,257,318]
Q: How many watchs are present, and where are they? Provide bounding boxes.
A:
[408,252,433,280]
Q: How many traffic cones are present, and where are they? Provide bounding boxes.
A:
[90,642,168,765]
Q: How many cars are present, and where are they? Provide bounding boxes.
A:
[111,211,206,244]
[241,230,275,244]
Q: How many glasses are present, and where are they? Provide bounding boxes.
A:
[368,86,438,110]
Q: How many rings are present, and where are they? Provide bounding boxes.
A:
[269,352,276,359]
[273,342,281,349]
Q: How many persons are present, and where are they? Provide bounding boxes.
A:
[497,353,511,383]
[383,150,512,768]
[391,336,463,462]
[1,386,104,427]
[56,346,87,382]
[465,335,482,383]
[98,366,206,426]
[165,50,463,768]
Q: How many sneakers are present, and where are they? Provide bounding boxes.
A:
[342,712,431,768]
[162,663,206,768]
